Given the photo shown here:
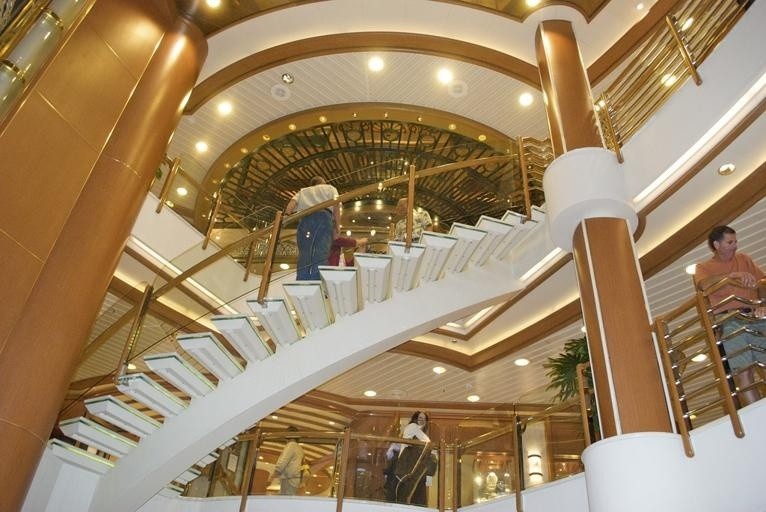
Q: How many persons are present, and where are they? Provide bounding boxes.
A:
[694,226,766,407]
[328,220,368,266]
[394,198,433,244]
[268,426,304,495]
[397,411,435,506]
[283,176,341,281]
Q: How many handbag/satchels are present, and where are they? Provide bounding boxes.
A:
[330,237,356,266]
[301,464,311,483]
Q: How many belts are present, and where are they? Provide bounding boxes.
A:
[720,308,755,314]
[324,207,332,216]
[411,237,419,242]
